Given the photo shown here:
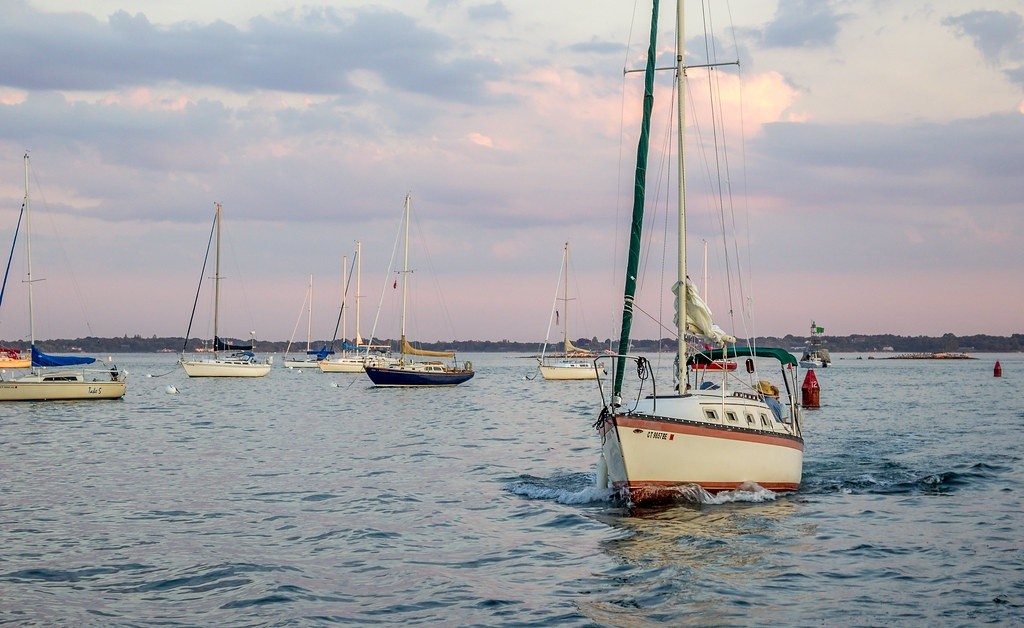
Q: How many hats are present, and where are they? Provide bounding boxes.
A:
[753,380,773,395]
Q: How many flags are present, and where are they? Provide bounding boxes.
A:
[816,327,825,334]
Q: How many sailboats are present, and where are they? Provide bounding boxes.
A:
[179,202,272,376]
[538,241,605,380]
[316,241,400,373]
[593,0,806,508]
[282,274,335,368]
[0,153,128,400]
[362,195,476,386]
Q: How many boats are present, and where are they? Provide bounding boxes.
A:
[800,321,831,368]
[0,344,31,368]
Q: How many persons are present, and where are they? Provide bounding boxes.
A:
[751,381,784,422]
[673,382,719,390]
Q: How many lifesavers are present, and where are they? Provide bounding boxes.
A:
[465,360,472,371]
